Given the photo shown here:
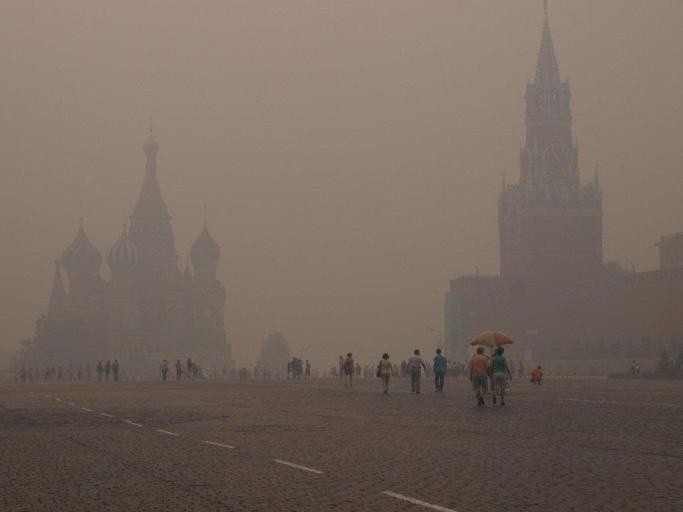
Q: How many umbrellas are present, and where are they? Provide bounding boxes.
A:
[468,329,512,353]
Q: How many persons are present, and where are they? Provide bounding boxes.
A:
[174,360,185,384]
[431,348,447,393]
[487,346,512,405]
[528,364,544,385]
[324,354,526,379]
[405,348,426,393]
[376,352,394,394]
[217,355,312,382]
[468,346,491,409]
[12,357,120,385]
[186,357,193,380]
[630,360,640,375]
[158,359,170,381]
[342,351,355,387]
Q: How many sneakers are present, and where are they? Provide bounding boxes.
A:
[477,396,484,406]
[493,395,504,405]
